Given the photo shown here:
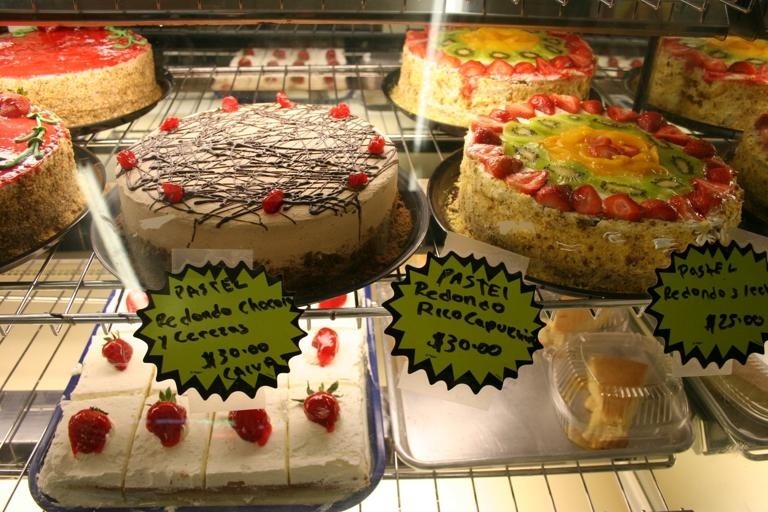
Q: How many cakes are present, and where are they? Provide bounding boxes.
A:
[733,339,765,392]
[568,353,647,448]
[116,90,416,304]
[647,35,768,130]
[538,295,619,349]
[2,26,164,130]
[0,89,88,267]
[37,284,371,509]
[444,91,744,293]
[599,56,646,81]
[391,24,597,130]
[730,114,767,221]
[210,45,352,98]
